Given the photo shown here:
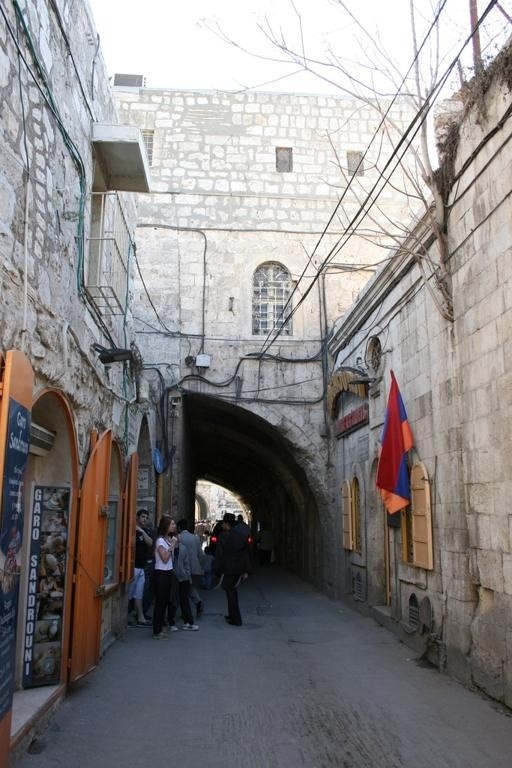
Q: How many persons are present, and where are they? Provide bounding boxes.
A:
[127,509,275,640]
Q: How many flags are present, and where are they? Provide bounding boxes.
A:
[375,372,415,515]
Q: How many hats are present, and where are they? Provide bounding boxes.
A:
[216,513,239,525]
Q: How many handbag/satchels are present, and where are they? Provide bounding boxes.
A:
[190,588,201,604]
[167,587,180,616]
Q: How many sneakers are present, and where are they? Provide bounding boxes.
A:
[196,602,204,619]
[225,615,243,626]
[126,614,199,640]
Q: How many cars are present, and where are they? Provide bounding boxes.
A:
[205,520,253,554]
